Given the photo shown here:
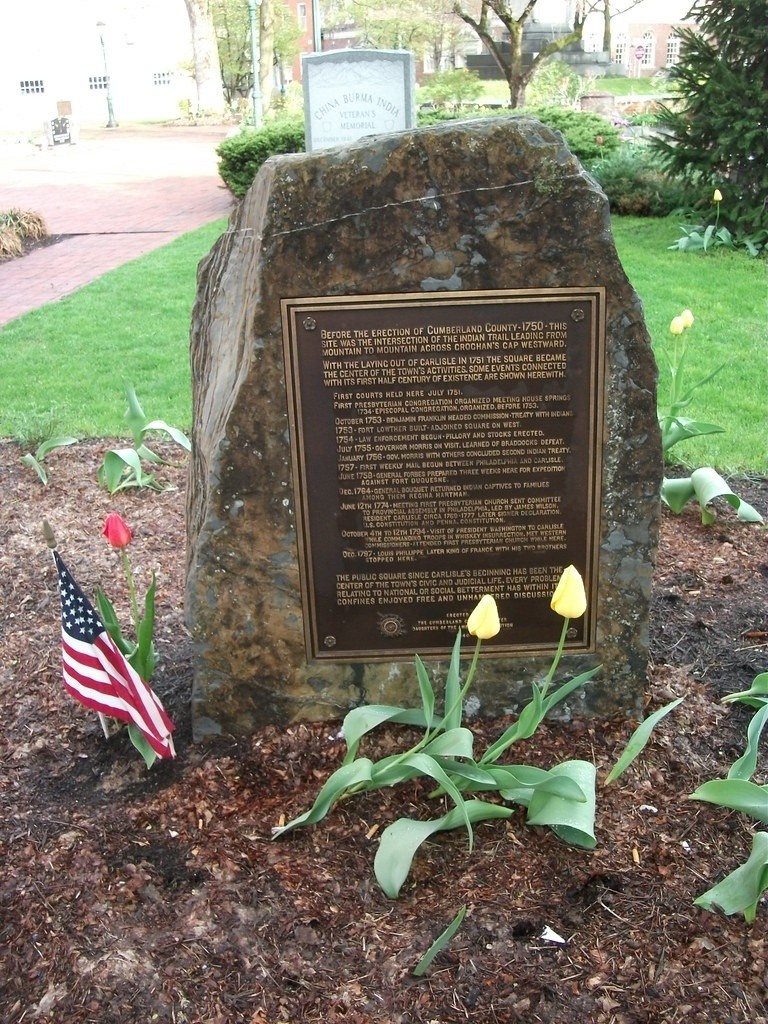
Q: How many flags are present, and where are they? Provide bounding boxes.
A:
[56,553,179,760]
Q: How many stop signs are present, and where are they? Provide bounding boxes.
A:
[635,46,644,59]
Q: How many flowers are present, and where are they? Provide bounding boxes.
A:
[651,296,724,457]
[82,511,177,770]
[272,552,628,905]
[668,181,736,253]
